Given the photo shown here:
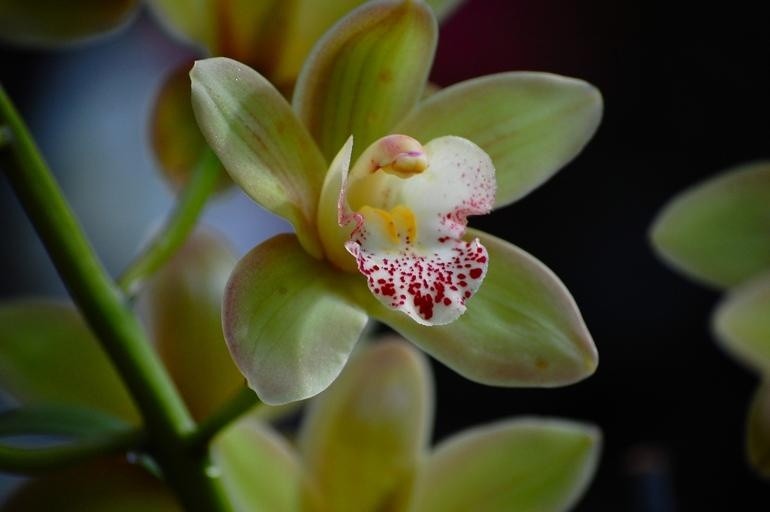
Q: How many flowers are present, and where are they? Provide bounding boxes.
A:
[0,1,770,511]
